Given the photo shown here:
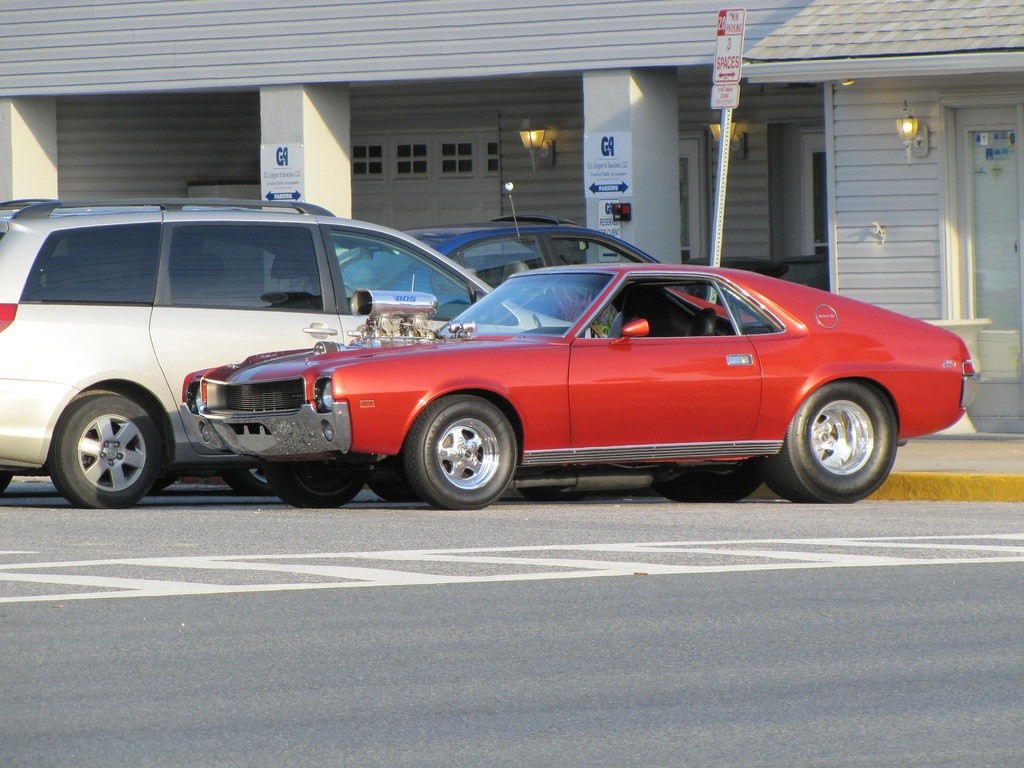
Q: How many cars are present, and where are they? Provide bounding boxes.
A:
[321,214,698,322]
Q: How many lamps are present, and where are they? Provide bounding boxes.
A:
[519,118,555,175]
[896,100,929,165]
[710,112,749,159]
[840,77,857,87]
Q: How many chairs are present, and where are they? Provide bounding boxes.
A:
[503,259,529,283]
[684,307,718,338]
[269,241,320,289]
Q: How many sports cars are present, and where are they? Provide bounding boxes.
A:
[178,262,984,513]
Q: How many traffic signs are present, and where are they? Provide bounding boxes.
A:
[713,9,743,85]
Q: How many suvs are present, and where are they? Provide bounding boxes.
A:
[1,197,602,512]
